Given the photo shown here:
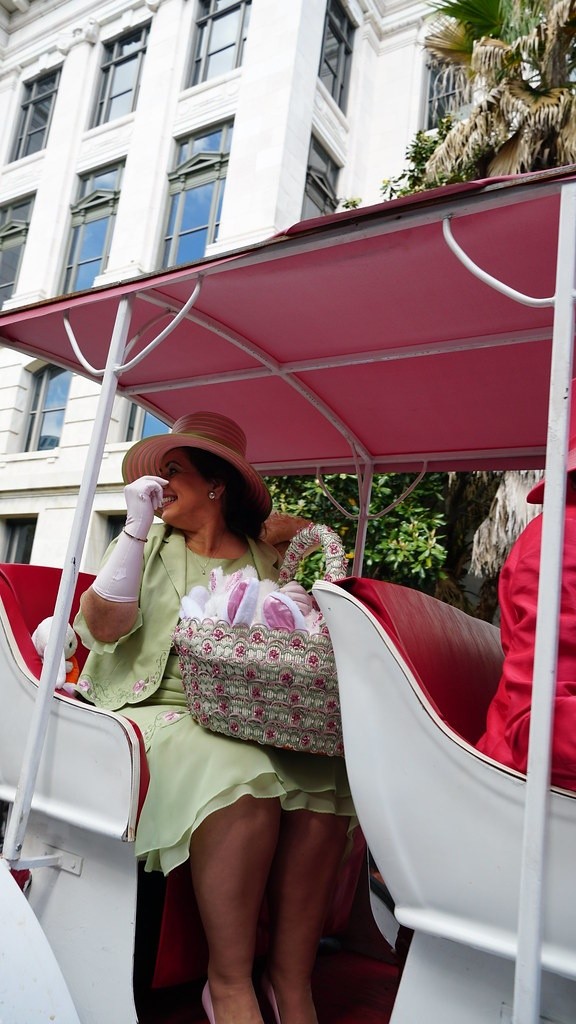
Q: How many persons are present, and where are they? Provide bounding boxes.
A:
[476,374,576,790]
[71,412,359,1024]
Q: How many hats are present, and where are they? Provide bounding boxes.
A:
[121,410,272,526]
[526,378,576,505]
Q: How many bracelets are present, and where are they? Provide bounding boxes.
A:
[123,527,148,542]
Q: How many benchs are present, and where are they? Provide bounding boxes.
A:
[0,563,210,1024]
[311,575,576,1024]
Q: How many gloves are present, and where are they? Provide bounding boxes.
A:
[92,475,170,602]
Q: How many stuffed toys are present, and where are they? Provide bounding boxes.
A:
[32,616,78,695]
[178,566,322,634]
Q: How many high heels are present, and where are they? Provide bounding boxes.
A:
[202,980,265,1024]
[261,970,318,1024]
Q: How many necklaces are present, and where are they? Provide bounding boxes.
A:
[185,528,225,574]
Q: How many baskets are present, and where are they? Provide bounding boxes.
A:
[173,522,349,757]
